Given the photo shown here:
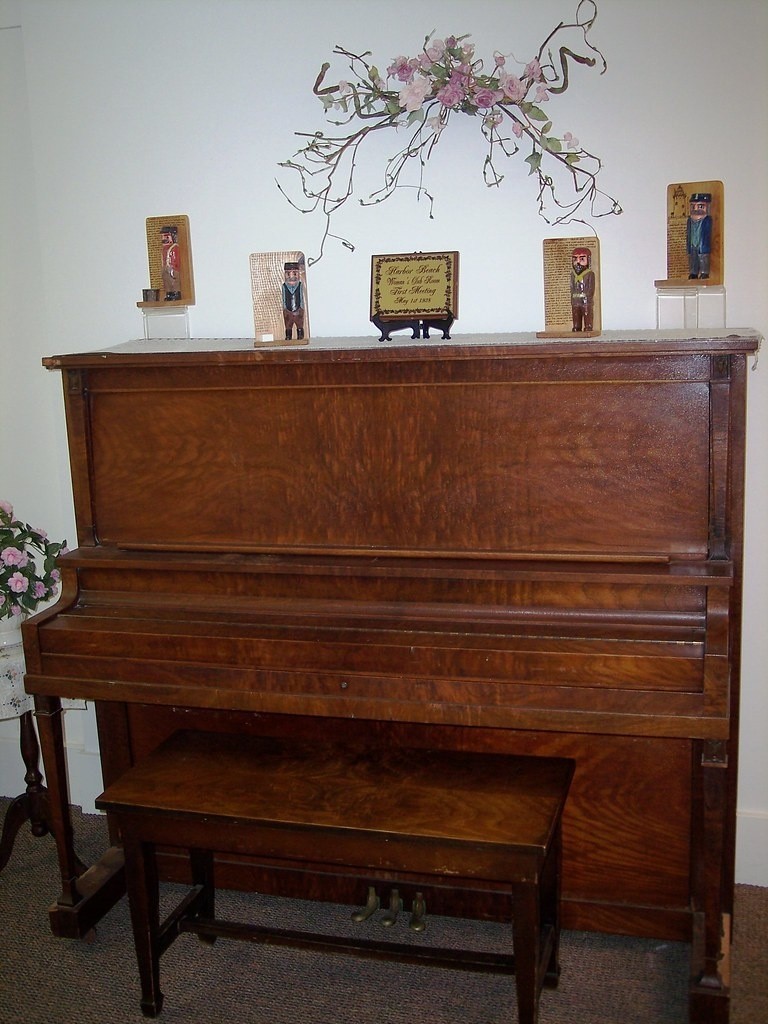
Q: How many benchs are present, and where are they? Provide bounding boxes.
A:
[94,727,578,1024]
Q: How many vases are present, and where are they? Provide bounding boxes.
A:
[0,611,25,646]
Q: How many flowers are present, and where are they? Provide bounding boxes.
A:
[0,501,72,622]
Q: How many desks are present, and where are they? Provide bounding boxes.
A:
[0,643,50,871]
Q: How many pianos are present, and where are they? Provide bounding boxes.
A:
[19,325,762,1024]
